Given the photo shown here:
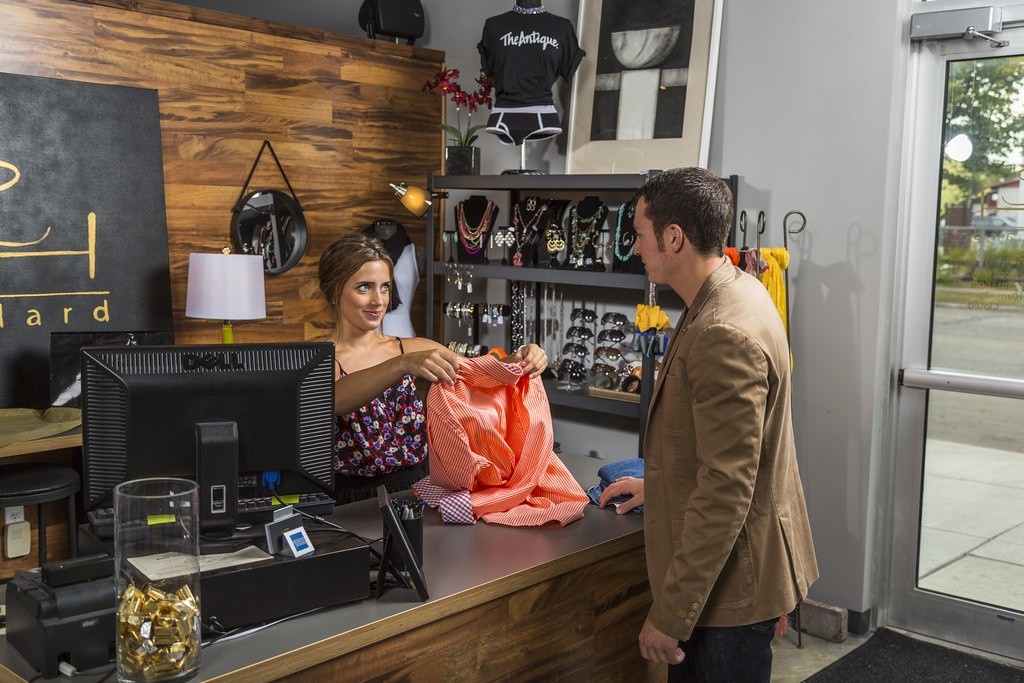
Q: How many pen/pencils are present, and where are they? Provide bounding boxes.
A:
[390,497,425,520]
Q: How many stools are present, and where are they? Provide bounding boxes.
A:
[0,464,80,628]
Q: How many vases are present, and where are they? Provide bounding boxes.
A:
[445,146,481,177]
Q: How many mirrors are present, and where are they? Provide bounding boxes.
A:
[230,189,308,276]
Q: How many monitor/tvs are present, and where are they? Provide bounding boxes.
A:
[81,342,336,555]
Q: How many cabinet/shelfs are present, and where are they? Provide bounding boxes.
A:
[425,176,738,491]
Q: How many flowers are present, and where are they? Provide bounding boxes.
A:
[420,67,496,146]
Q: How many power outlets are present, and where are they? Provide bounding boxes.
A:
[5,506,24,525]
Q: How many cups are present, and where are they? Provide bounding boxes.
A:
[114,478,201,683]
[383,515,424,571]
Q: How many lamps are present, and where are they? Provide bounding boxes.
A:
[185,247,266,344]
[389,181,449,215]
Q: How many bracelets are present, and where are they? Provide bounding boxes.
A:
[449,340,482,358]
[446,300,503,320]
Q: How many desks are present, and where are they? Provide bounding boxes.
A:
[0,424,82,456]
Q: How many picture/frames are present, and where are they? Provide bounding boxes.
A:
[565,0,724,175]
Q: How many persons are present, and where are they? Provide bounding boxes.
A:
[631,163,822,683]
[311,233,546,509]
[478,0,586,148]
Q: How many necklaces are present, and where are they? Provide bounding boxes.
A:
[569,202,605,265]
[614,204,635,261]
[456,198,496,253]
[512,201,546,265]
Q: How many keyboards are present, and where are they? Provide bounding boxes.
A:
[86,493,336,540]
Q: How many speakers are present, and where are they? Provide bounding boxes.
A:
[358,0,425,39]
